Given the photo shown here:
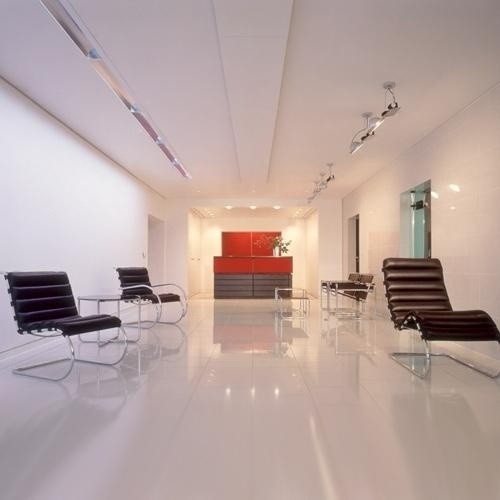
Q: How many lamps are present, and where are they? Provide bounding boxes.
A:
[347,79,401,158]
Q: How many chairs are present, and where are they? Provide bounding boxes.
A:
[381,257,499,386]
[2,261,192,383]
[320,272,375,320]
